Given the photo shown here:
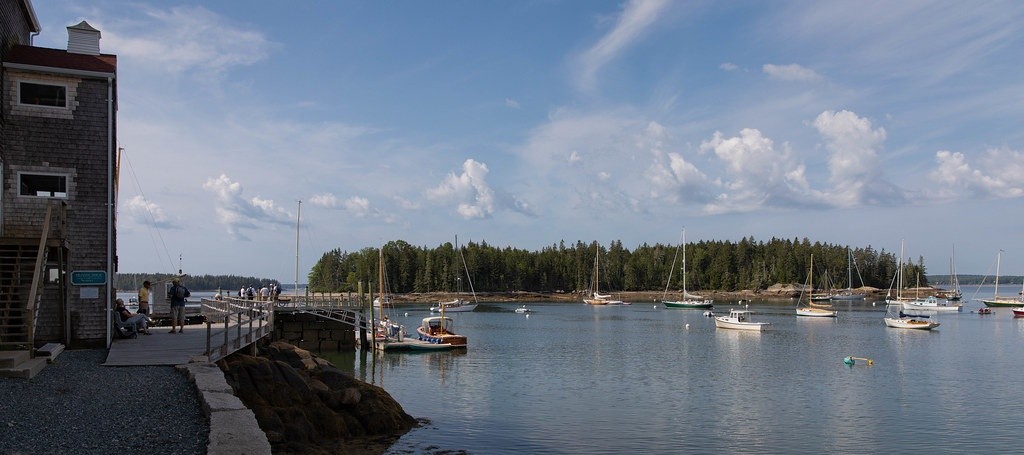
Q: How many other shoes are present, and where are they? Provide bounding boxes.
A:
[149,322,156,326]
[168,330,176,334]
[179,330,183,333]
[144,331,152,335]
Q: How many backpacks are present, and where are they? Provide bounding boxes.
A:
[276,286,281,295]
[247,288,252,295]
[173,286,185,300]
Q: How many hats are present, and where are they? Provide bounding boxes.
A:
[249,284,252,287]
[172,278,179,283]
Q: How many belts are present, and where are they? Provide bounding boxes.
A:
[143,302,148,303]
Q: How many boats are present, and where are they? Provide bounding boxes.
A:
[715,309,774,331]
[886,298,936,306]
[583,292,622,305]
[903,303,963,311]
[416,316,468,348]
[129,296,138,310]
[515,307,531,313]
[982,299,1024,307]
[1013,307,1024,318]
[796,307,839,317]
[430,297,478,312]
[664,300,714,308]
[833,288,871,300]
[807,293,830,300]
[885,317,939,330]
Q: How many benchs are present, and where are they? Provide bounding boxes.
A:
[115,311,138,338]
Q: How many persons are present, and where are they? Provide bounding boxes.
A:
[169,278,190,333]
[116,298,158,335]
[240,283,277,302]
[138,280,151,333]
[215,293,222,300]
